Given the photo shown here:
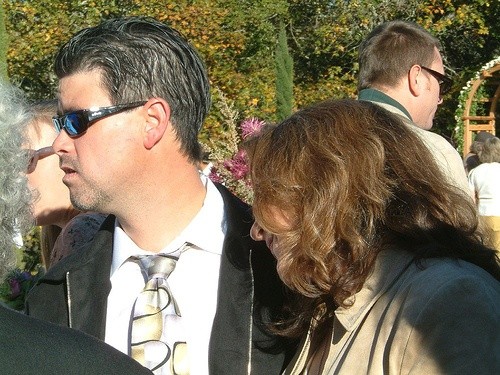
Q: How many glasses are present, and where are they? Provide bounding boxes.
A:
[22,146,55,175]
[420,65,454,95]
[51,101,147,138]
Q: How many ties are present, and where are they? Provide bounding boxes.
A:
[128,242,193,375]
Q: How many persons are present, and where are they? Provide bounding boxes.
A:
[353,21,473,208]
[21,18,310,375]
[469,138,500,253]
[466,131,494,168]
[9,100,96,278]
[0,301,154,375]
[249,98,499,374]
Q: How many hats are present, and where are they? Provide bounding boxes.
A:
[474,132,495,142]
